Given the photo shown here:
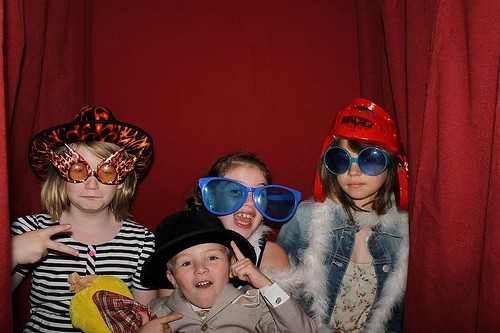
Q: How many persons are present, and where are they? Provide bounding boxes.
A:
[10,105,155,333]
[158,152,291,299]
[135,210,320,333]
[274,98,409,333]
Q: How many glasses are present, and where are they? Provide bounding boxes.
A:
[325,146,390,177]
[199,177,303,223]
[50,144,134,184]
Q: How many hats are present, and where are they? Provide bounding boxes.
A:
[27,103,154,186]
[139,208,257,290]
[314,98,409,211]
